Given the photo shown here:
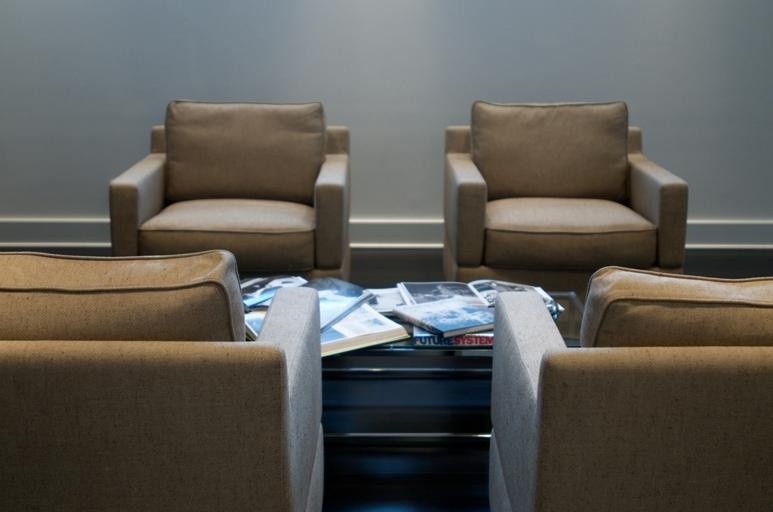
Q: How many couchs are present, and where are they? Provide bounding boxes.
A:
[3,251,323,508]
[110,99,353,283]
[483,265,770,506]
[442,102,689,298]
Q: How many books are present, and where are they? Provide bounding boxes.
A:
[392,279,565,346]
[239,275,411,358]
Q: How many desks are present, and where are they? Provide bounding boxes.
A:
[240,276,587,512]
